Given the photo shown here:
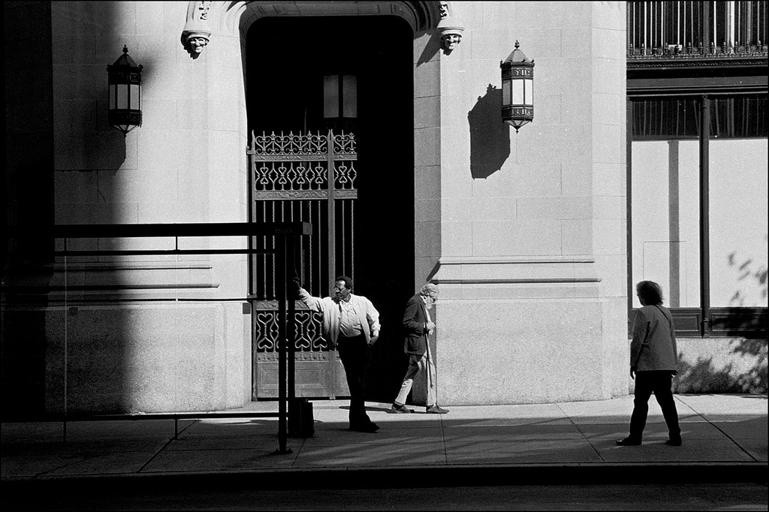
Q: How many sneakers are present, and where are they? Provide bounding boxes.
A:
[666,436,682,445]
[616,437,642,445]
[392,401,414,413]
[427,405,449,414]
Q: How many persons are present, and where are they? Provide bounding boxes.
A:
[292,276,384,431]
[615,282,683,446]
[390,282,449,414]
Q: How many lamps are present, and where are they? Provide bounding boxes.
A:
[105,41,145,140]
[499,39,535,136]
